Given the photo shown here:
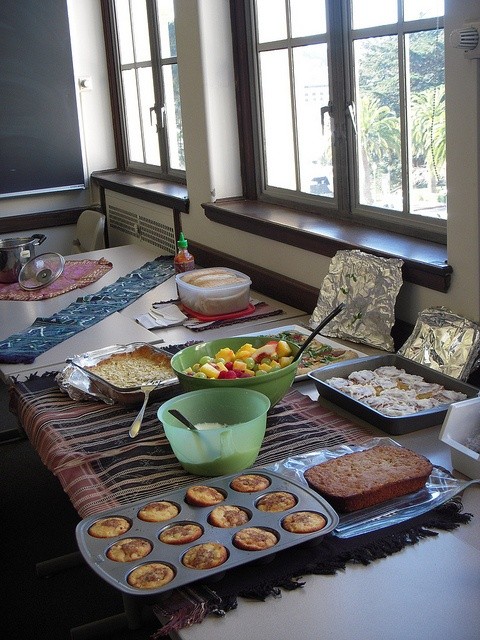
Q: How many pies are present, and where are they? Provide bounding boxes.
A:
[181,269,249,316]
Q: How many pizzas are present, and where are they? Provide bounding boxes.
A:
[258,330,358,376]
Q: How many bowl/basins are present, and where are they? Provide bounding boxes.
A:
[157,387,271,475]
[170,337,302,410]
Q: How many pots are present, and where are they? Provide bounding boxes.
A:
[0,234,47,284]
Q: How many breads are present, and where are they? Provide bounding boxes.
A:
[303,444,434,513]
[283,512,327,532]
[230,474,270,492]
[128,563,175,590]
[88,517,131,538]
[107,537,152,562]
[186,485,224,507]
[233,527,277,551]
[138,502,179,522]
[255,491,296,512]
[159,525,203,543]
[209,506,249,528]
[183,543,227,569]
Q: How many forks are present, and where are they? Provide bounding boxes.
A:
[129,379,161,440]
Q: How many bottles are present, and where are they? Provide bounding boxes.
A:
[174,232,196,295]
